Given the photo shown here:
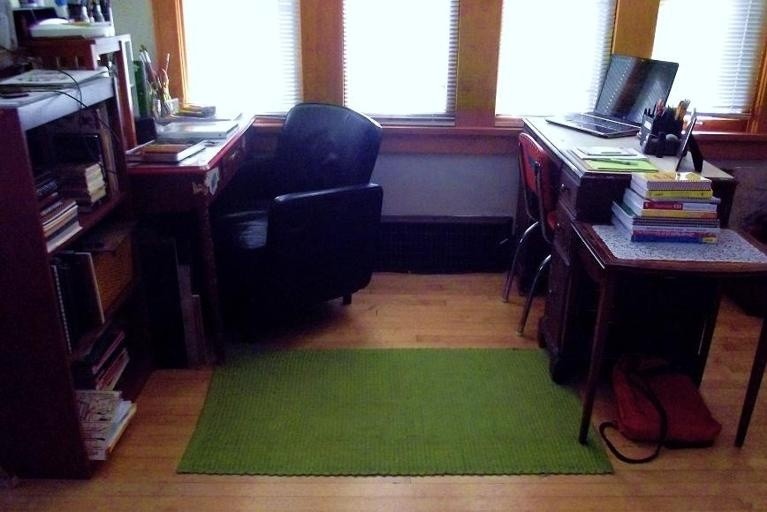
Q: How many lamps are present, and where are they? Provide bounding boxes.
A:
[503,133,556,336]
[214,102,383,344]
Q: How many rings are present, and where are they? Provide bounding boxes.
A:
[644,99,657,118]
[592,158,634,165]
[675,98,691,121]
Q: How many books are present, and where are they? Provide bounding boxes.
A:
[92,227,134,313]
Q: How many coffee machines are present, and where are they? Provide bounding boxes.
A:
[656,99,665,117]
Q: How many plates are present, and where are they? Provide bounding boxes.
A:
[0,1,141,479]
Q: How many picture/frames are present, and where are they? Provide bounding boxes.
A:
[515,116,738,392]
[560,220,767,446]
[128,114,256,371]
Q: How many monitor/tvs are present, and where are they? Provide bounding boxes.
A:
[66,324,140,461]
[609,170,723,246]
[33,159,108,254]
[124,119,241,164]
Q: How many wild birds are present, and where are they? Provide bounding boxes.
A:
[545,51,680,139]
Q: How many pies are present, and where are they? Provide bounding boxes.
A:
[138,44,173,118]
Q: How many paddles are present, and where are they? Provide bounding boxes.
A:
[175,349,613,476]
